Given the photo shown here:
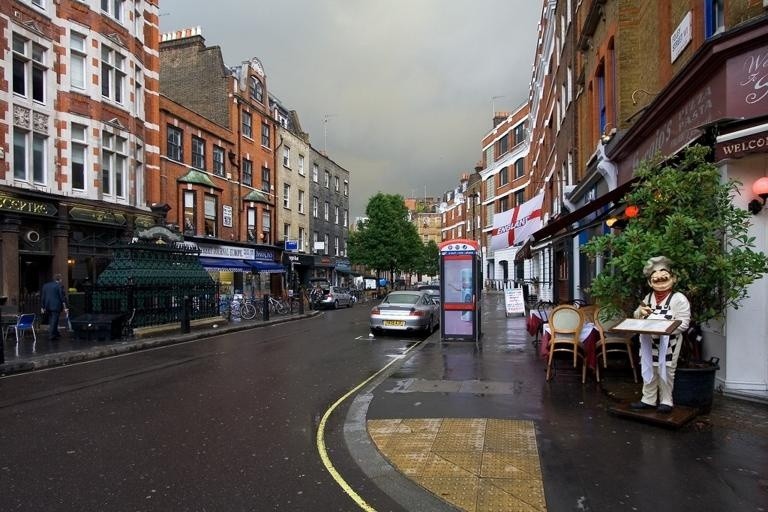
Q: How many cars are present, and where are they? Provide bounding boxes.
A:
[369,281,441,336]
[308,284,356,311]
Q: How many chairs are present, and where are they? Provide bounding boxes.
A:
[534,298,638,386]
[5,312,38,343]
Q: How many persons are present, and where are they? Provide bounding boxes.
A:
[41,272,69,341]
[306,283,323,312]
[633,256,690,413]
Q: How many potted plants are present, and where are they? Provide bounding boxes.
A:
[580,142,768,421]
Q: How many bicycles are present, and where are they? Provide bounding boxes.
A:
[219,296,303,320]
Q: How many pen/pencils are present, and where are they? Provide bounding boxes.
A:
[638,299,651,314]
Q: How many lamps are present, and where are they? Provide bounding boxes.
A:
[748,178,768,214]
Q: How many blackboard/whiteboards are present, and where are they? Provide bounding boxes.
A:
[505,288,525,313]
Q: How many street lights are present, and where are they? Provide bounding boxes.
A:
[468,188,479,241]
